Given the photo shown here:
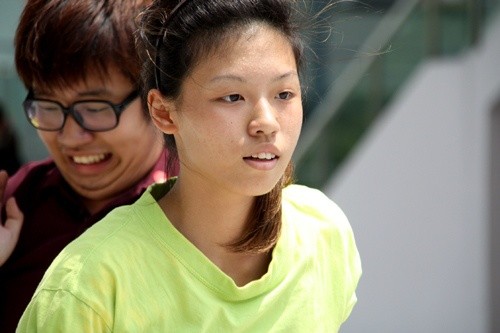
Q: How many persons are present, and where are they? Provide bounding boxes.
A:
[16,1,364,332]
[0,0,181,332]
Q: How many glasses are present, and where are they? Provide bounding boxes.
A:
[23,89,141,132]
